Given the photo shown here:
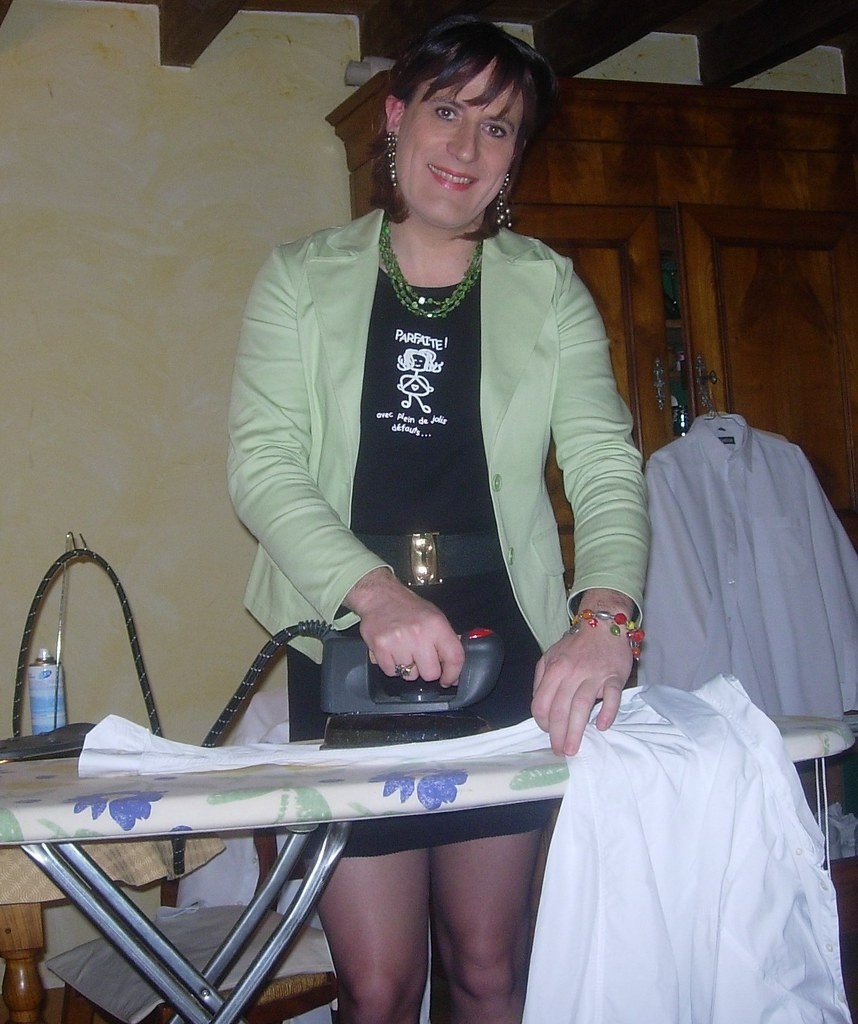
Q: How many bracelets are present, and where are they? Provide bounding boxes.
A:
[569,607,643,662]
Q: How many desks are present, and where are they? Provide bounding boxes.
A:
[0,831,226,1024]
[1,714,857,1024]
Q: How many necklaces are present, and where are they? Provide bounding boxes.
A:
[379,225,481,319]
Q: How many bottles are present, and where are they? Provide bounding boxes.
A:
[679,351,686,376]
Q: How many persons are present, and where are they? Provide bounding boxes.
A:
[225,15,655,1024]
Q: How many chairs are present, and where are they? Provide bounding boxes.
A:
[62,825,336,1024]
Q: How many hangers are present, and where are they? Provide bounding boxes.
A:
[702,375,747,429]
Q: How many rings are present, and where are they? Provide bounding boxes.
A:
[396,662,415,676]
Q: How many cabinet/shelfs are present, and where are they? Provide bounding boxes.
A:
[321,69,858,934]
